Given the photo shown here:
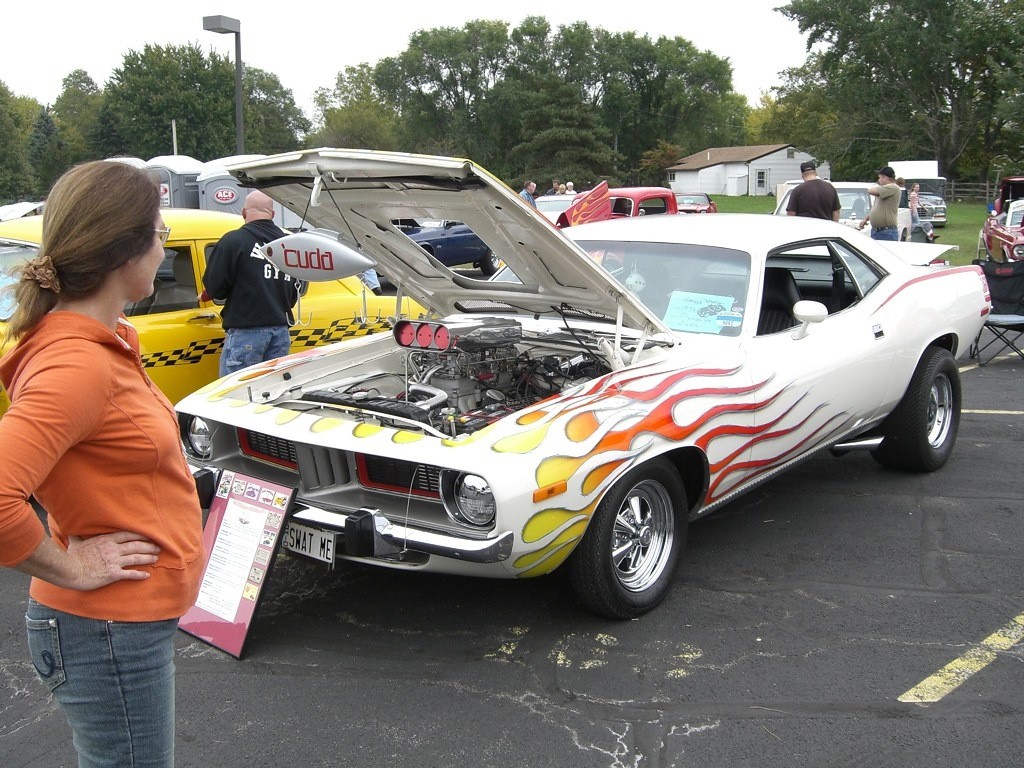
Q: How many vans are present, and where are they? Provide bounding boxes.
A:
[768,182,911,243]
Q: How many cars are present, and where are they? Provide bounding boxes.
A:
[671,192,718,213]
[982,175,1024,263]
[554,179,689,271]
[389,219,499,276]
[0,212,439,418]
[174,146,994,620]
[907,191,947,225]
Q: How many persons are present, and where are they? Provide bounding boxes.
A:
[519,181,536,208]
[895,178,920,225]
[364,268,382,296]
[786,162,841,222]
[546,179,578,195]
[859,167,902,241]
[0,162,207,768]
[202,190,297,378]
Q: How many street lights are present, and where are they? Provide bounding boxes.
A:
[202,15,245,154]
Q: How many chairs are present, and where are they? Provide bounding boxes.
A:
[123,277,161,317]
[852,198,866,213]
[147,251,199,314]
[612,199,627,214]
[710,267,805,335]
[969,259,1024,368]
[635,263,674,320]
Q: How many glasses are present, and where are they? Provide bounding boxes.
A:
[155,227,171,244]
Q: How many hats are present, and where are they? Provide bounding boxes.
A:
[800,161,815,172]
[874,166,895,178]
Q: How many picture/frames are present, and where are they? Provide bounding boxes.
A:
[176,467,298,662]
